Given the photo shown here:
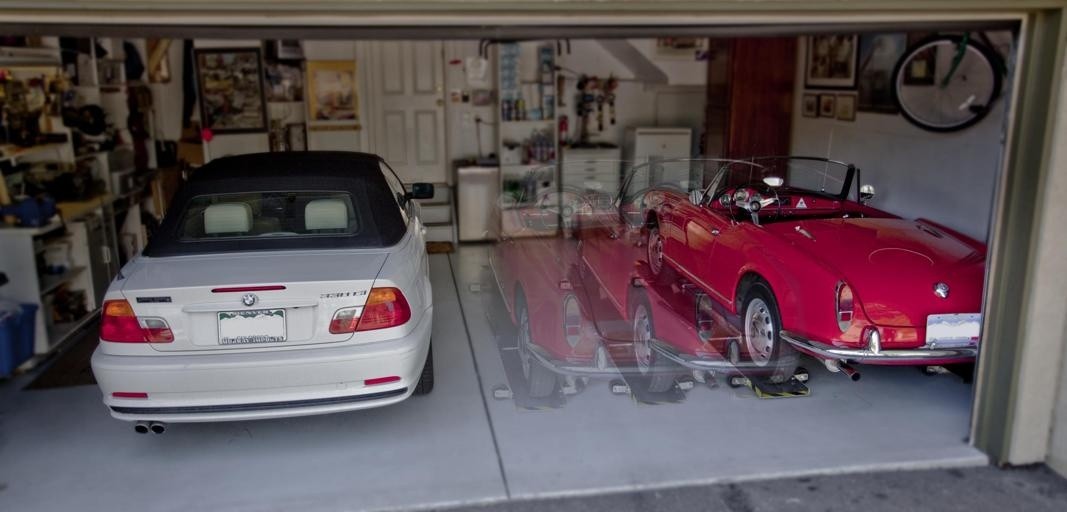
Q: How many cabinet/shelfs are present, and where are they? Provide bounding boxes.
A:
[495,40,561,239]
[561,140,621,240]
[0,25,189,361]
[624,124,695,198]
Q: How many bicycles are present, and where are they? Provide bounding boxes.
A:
[892,26,1019,135]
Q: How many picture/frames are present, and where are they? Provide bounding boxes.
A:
[801,37,858,123]
[192,45,271,138]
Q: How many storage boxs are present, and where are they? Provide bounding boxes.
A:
[0,298,37,381]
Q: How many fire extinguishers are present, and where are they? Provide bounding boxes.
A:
[558,114,568,147]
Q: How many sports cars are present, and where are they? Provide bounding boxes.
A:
[490,152,993,404]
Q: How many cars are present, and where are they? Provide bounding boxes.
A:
[91,147,438,438]
